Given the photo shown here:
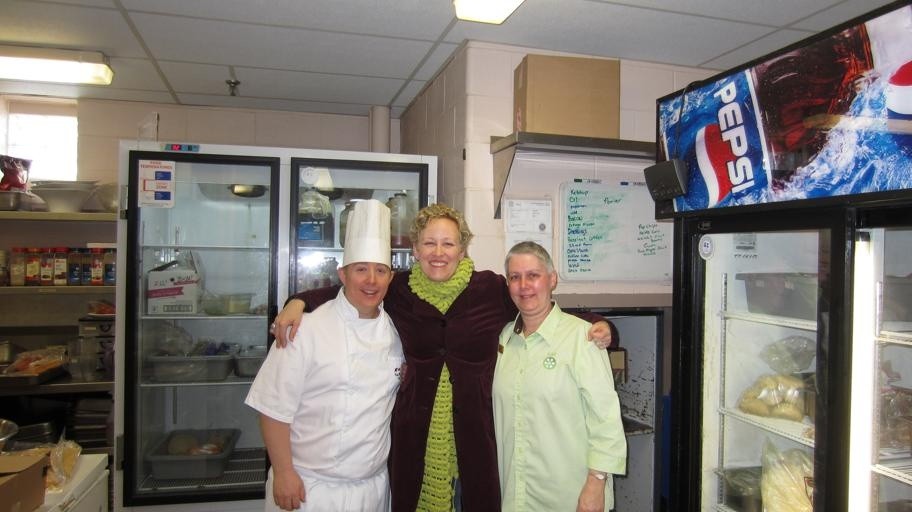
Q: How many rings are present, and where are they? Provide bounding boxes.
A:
[271,325,273,333]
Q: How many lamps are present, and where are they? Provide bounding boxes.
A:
[0,41,114,87]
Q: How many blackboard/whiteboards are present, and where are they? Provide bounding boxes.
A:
[556,182,675,285]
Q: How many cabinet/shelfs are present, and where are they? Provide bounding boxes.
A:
[1,211,118,466]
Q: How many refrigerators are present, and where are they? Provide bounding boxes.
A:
[116,138,437,512]
[654,189,912,512]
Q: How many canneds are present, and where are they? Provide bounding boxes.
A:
[9,247,117,287]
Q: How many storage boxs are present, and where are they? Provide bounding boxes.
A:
[735,271,912,322]
[144,429,241,480]
[203,292,256,315]
[234,345,270,378]
[79,354,112,383]
[713,465,763,512]
[512,53,620,139]
[78,337,114,354]
[146,343,241,383]
[145,251,207,316]
[0,449,51,512]
[78,318,116,337]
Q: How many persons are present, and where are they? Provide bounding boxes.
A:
[244,200,407,512]
[491,242,629,512]
[268,201,620,512]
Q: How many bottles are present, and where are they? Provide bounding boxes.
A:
[9,246,115,286]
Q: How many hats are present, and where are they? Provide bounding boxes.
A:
[337,198,396,271]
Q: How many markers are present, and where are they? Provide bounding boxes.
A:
[574,178,601,184]
[622,181,648,184]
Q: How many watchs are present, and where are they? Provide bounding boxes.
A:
[590,472,610,482]
